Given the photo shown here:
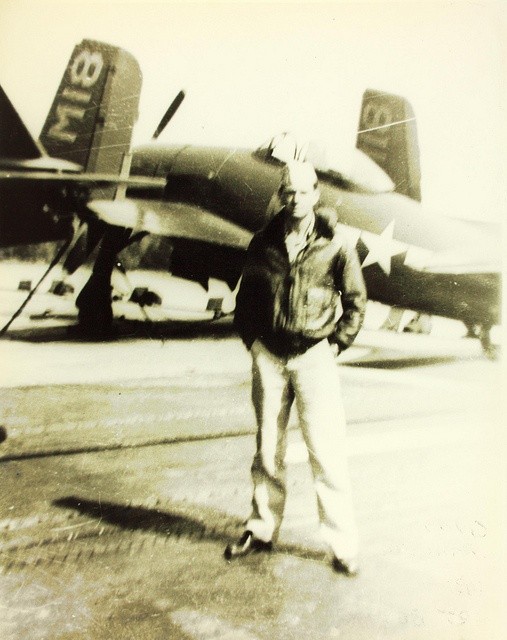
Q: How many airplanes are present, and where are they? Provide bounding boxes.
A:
[0,38,503,350]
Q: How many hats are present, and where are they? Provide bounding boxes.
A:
[282,160,317,186]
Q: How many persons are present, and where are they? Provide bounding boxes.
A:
[222,159,367,576]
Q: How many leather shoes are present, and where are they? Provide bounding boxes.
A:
[225,531,273,557]
[334,555,363,577]
